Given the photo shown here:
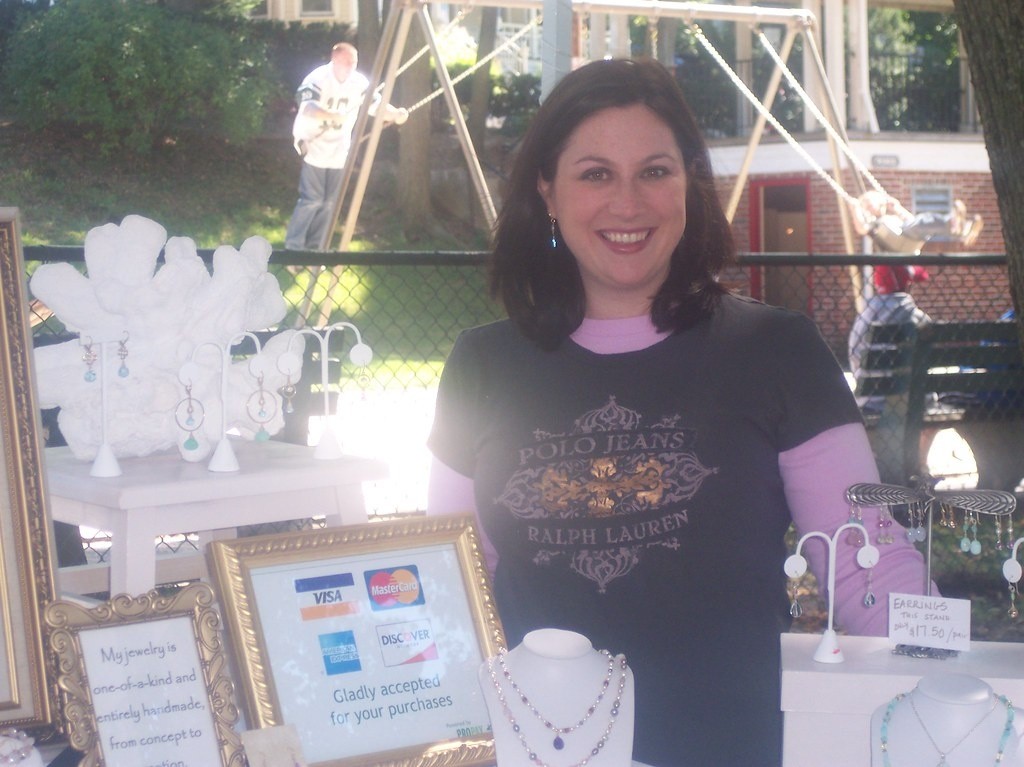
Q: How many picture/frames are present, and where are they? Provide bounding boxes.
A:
[0,208,74,742]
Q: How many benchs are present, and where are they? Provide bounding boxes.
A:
[850,319,1024,425]
[26,325,347,453]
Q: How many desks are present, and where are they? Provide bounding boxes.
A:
[35,437,411,612]
[781,633,1024,767]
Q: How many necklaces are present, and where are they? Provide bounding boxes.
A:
[880,692,1016,767]
[911,692,998,766]
[487,648,627,766]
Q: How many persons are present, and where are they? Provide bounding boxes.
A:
[285,42,408,270]
[423,60,951,766]
[848,264,933,416]
[842,191,983,257]
